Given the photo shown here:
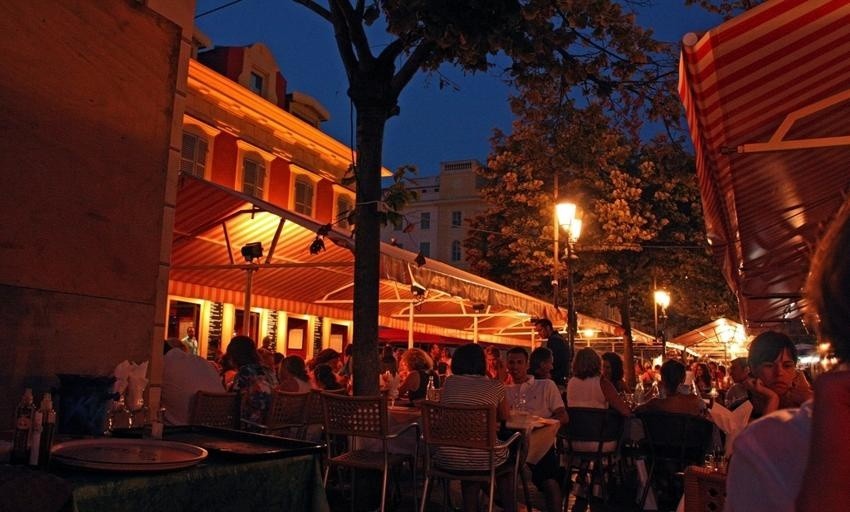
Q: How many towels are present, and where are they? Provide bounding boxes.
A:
[114,360,148,413]
[388,372,399,398]
[707,401,753,458]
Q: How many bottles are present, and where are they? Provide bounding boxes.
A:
[10,388,35,466]
[426,377,436,400]
[28,394,57,471]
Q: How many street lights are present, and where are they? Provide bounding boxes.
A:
[654,287,670,360]
[654,284,673,370]
[554,193,585,358]
[553,202,583,351]
[717,318,731,365]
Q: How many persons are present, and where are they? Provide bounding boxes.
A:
[181,326,197,356]
[485,345,507,380]
[379,346,396,375]
[273,353,284,378]
[207,341,225,364]
[727,357,754,410]
[159,339,227,426]
[567,346,631,453]
[220,355,238,390]
[728,201,849,511]
[338,343,352,375]
[227,335,280,433]
[307,348,339,382]
[258,336,276,367]
[634,360,704,512]
[434,343,510,512]
[270,356,321,440]
[396,348,407,360]
[396,347,440,408]
[602,352,628,393]
[720,330,798,452]
[257,349,275,373]
[527,347,554,380]
[502,347,569,512]
[314,364,342,391]
[429,344,452,386]
[641,362,660,393]
[535,319,572,385]
[695,362,726,393]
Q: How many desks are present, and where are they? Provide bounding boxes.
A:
[362,407,559,512]
[1,423,329,511]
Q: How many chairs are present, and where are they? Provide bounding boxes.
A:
[635,411,727,512]
[322,392,421,512]
[303,388,349,439]
[191,390,250,423]
[240,389,313,434]
[558,406,626,512]
[420,400,523,512]
[683,465,726,512]
